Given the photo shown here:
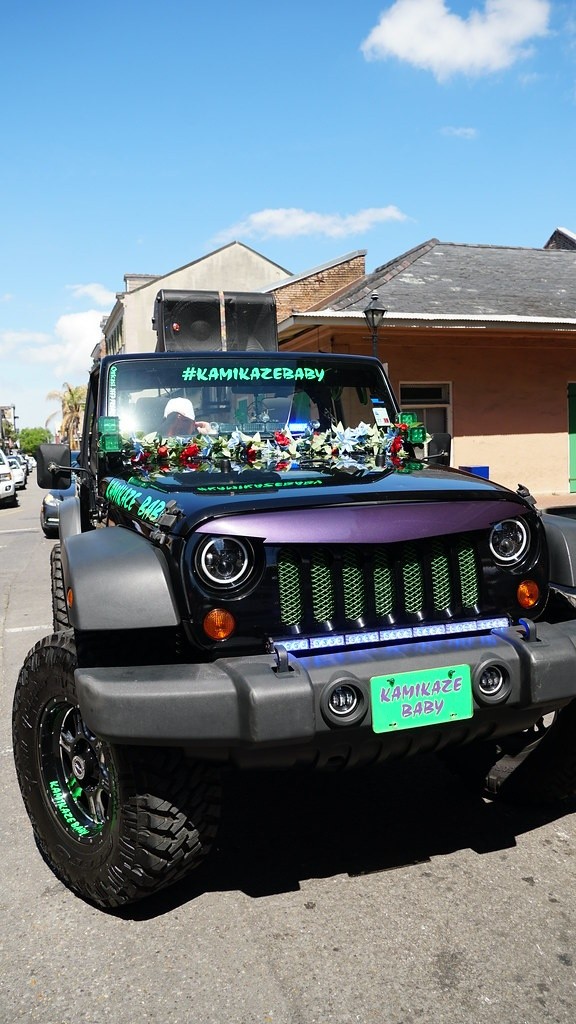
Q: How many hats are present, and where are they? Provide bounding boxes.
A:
[163,397,195,421]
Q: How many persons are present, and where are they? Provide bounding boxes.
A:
[143,397,219,440]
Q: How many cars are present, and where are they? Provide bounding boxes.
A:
[8,459,26,490]
[0,449,17,509]
[29,456,37,467]
[7,455,29,476]
[41,450,82,538]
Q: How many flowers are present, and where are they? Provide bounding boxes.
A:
[111,420,410,482]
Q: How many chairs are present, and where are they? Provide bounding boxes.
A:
[261,397,294,421]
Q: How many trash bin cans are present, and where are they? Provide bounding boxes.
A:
[458,465,489,480]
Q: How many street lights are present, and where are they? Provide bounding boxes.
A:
[361,292,388,358]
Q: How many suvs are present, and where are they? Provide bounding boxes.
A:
[12,347,576,903]
